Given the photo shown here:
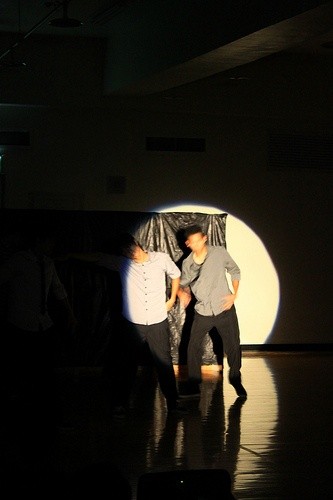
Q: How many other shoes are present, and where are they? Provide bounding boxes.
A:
[229,379,246,397]
[179,384,200,397]
[114,407,153,418]
[167,398,179,412]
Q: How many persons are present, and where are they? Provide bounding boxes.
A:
[75,232,192,415]
[180,224,248,397]
[1,224,82,416]
